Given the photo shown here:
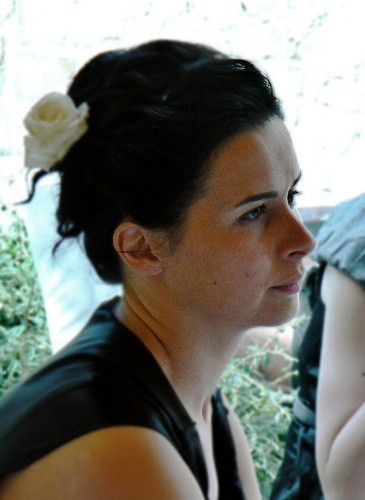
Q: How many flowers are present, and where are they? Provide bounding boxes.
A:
[22,90,92,168]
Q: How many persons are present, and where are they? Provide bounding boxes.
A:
[271,193,364,500]
[1,39,316,500]
